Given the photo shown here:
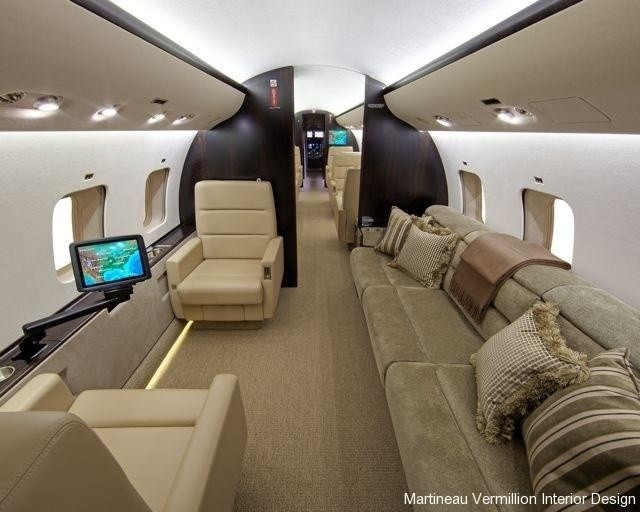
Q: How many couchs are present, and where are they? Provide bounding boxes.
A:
[348,205,640,512]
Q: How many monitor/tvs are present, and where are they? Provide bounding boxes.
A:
[327,129,348,145]
[69,233,153,294]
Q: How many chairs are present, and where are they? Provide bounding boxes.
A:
[0,373,246,512]
[295,145,303,199]
[325,143,362,243]
[166,178,284,328]
